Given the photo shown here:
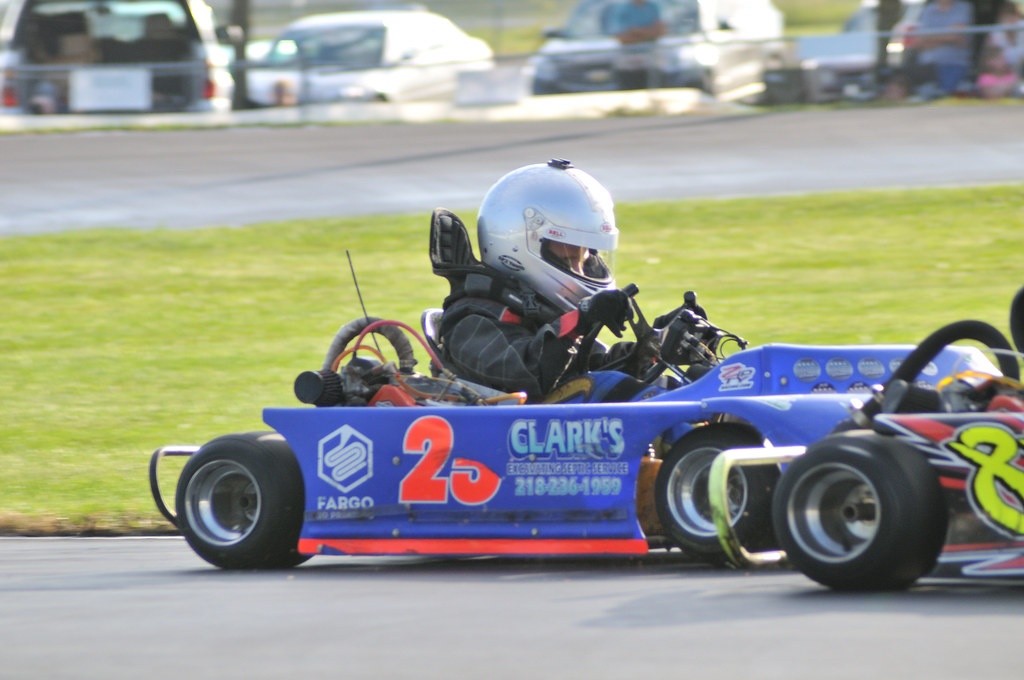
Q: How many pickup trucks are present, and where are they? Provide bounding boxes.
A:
[1,0,238,112]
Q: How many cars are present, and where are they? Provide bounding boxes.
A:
[241,8,495,102]
[516,0,789,102]
[759,2,938,100]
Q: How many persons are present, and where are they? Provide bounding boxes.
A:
[901,0,1024,97]
[438,159,708,402]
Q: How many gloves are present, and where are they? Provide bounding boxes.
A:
[652,303,707,329]
[579,290,634,338]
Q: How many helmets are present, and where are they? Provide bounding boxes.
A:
[478,159,618,314]
